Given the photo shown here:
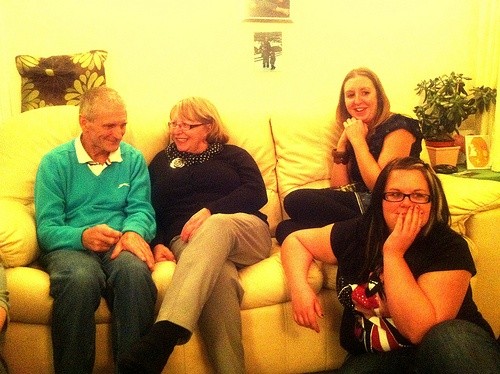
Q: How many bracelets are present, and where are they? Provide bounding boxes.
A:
[332,149,350,165]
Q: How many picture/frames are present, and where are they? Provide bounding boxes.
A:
[464,135,493,170]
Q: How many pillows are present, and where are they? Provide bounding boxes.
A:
[16,51,107,113]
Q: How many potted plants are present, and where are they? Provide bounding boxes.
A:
[414,72,497,150]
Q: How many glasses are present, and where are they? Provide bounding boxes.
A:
[169,121,202,130]
[382,192,431,204]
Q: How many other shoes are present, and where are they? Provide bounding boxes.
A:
[117,329,174,374]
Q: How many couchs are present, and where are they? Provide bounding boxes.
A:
[0,105,500,374]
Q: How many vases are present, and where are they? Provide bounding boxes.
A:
[425,145,462,167]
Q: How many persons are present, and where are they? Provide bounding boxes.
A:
[116,97,273,374]
[281,156,500,374]
[35,86,158,374]
[0,256,11,374]
[261,36,279,70]
[275,67,423,246]
